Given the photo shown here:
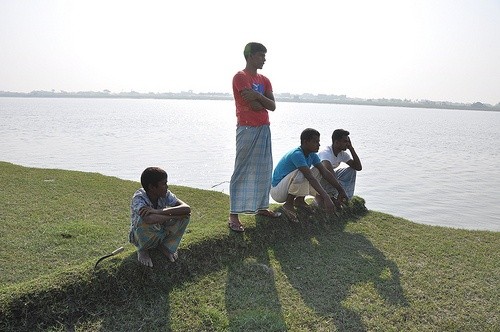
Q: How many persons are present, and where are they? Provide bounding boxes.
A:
[129,167,191,267]
[228,42,282,232]
[308,129,363,210]
[270,128,350,223]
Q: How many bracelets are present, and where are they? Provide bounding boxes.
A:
[160,208,163,214]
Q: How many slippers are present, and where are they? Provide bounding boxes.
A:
[294,205,314,217]
[283,207,300,222]
[227,216,245,231]
[257,209,282,218]
